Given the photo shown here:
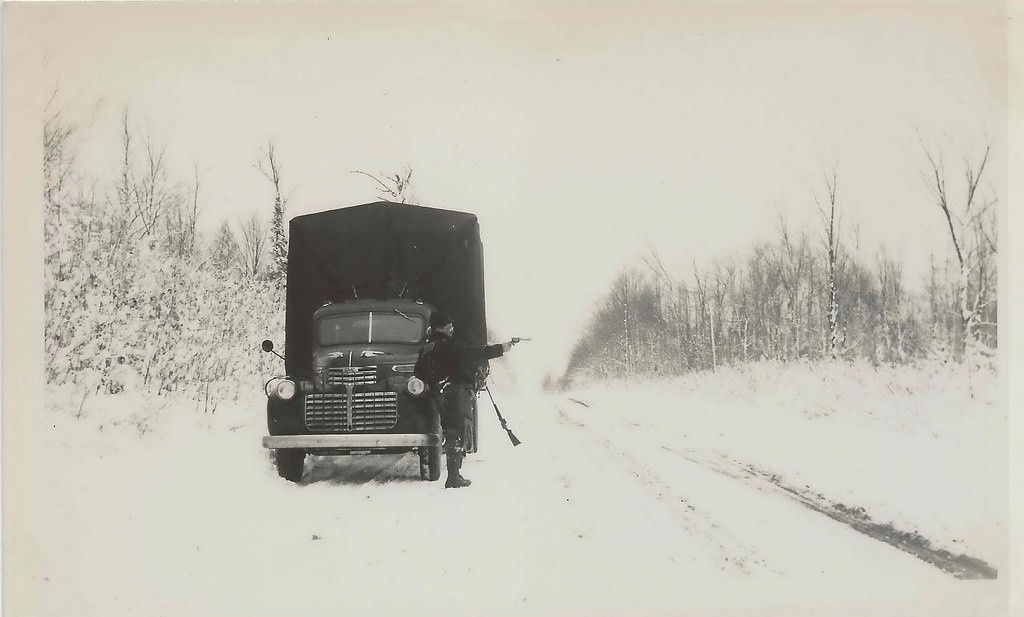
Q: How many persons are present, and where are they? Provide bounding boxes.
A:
[408,310,517,490]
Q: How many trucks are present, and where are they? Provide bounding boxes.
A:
[262,201,488,481]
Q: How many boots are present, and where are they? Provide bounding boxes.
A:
[445,454,472,489]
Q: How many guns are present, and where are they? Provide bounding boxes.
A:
[510,337,533,344]
[486,385,522,446]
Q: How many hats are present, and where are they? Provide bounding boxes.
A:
[430,311,452,330]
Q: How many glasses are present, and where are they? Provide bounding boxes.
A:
[449,327,454,331]
